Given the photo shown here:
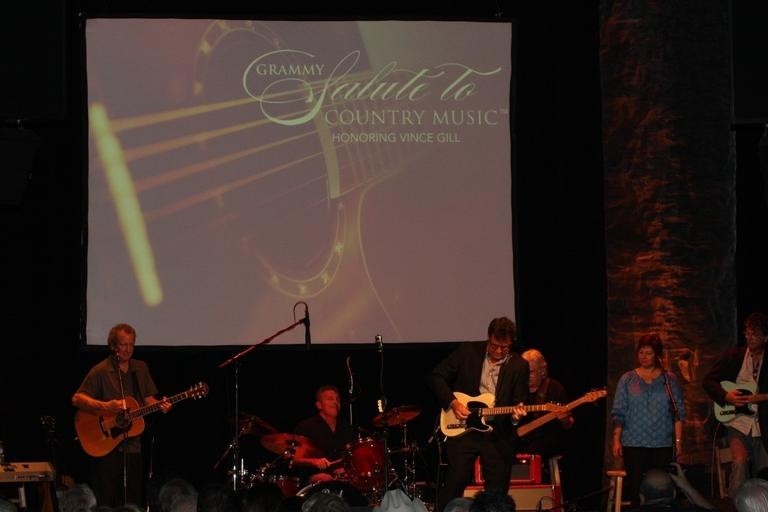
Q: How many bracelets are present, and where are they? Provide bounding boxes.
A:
[676,439,683,442]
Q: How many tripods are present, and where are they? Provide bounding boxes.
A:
[386,423,435,503]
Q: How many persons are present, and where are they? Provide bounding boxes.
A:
[291,385,351,487]
[0,460,768,512]
[70,322,173,511]
[431,317,534,511]
[703,311,767,493]
[610,333,688,511]
[499,349,575,484]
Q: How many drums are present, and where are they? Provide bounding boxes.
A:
[291,480,368,512]
[267,474,302,497]
[340,436,395,493]
[391,448,427,489]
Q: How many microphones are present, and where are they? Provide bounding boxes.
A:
[305,307,312,353]
[375,335,383,353]
[113,348,120,357]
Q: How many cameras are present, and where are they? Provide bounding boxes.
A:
[667,464,678,476]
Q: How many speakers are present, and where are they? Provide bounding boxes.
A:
[460,485,563,512]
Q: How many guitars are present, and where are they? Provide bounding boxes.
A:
[516,386,608,437]
[74,382,209,458]
[714,381,768,422]
[440,391,569,438]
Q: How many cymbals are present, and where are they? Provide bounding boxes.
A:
[231,408,278,435]
[261,433,322,458]
[373,406,421,428]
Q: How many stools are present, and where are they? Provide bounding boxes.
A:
[604,470,632,512]
